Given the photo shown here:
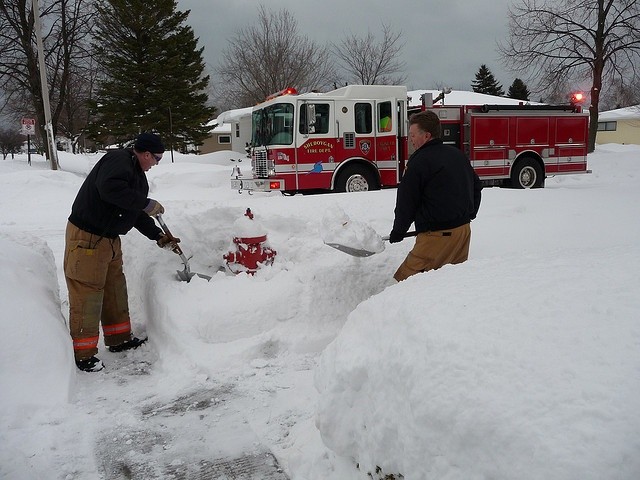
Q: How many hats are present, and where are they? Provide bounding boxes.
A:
[134,132,164,153]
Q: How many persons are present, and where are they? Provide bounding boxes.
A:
[64,133,180,372]
[390,110,483,281]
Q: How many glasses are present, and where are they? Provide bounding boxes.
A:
[144,149,162,161]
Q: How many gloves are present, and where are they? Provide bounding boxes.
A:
[156,232,180,254]
[390,230,405,243]
[142,199,164,218]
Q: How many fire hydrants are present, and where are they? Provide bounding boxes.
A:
[223,208,276,275]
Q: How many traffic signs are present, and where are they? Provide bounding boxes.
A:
[21,118,35,136]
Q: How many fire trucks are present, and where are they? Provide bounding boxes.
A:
[232,85,592,195]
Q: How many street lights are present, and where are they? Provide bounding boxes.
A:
[170,106,181,163]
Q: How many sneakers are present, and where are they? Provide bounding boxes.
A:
[75,356,105,372]
[109,337,148,352]
